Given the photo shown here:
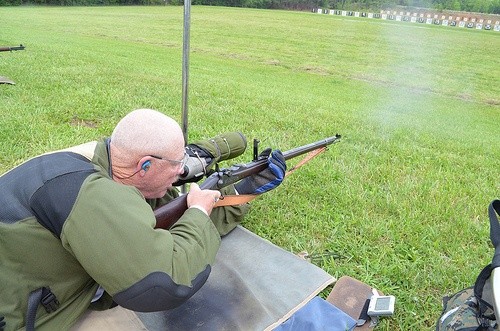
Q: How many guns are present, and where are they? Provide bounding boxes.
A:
[0,44,26,51]
[152,130,341,233]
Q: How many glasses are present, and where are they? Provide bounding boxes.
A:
[144,149,189,171]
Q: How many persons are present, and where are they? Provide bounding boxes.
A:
[0,109,287,331]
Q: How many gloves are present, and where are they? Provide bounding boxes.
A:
[234,148,287,195]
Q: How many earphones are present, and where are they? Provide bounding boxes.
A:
[142,160,151,172]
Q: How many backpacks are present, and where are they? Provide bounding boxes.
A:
[437,199,500,331]
